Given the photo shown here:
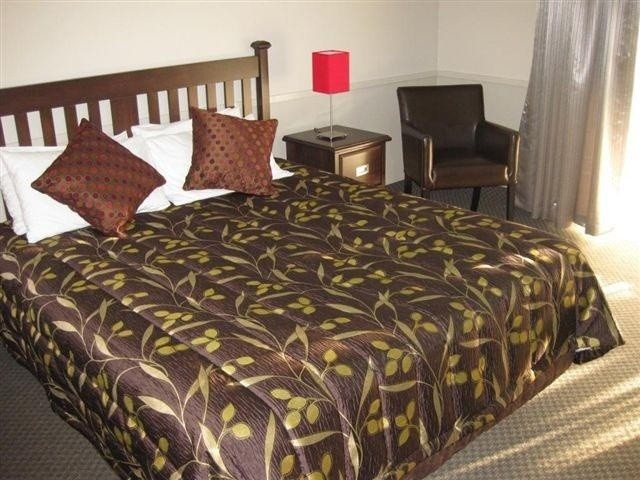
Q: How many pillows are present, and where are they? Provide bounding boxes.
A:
[1,118,173,245]
[128,106,294,209]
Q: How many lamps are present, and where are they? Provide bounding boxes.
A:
[311,49,350,142]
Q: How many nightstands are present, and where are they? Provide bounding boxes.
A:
[283,125,392,190]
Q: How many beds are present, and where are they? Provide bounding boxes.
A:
[0,42,624,480]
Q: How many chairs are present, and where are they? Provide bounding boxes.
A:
[396,83,520,222]
